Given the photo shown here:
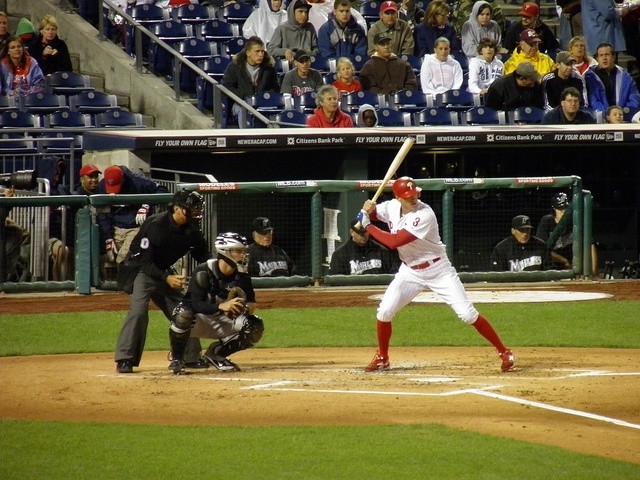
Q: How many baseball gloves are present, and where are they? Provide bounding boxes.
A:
[225,288,248,319]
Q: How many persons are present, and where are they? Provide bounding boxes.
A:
[605,105,624,123]
[67,164,106,254]
[488,214,547,272]
[503,29,558,85]
[305,84,354,128]
[30,156,72,280]
[244,216,306,276]
[485,63,545,109]
[580,0,627,65]
[95,165,168,265]
[114,187,214,373]
[0,10,12,55]
[0,35,44,96]
[356,103,382,127]
[419,36,464,100]
[461,1,503,57]
[28,14,72,76]
[221,36,281,128]
[540,51,588,110]
[266,0,320,64]
[167,231,265,374]
[100,0,191,47]
[328,218,399,274]
[360,32,418,95]
[242,0,288,52]
[397,0,425,32]
[567,36,599,78]
[584,43,640,122]
[413,0,463,56]
[301,0,368,37]
[356,176,515,372]
[503,2,561,52]
[543,86,597,124]
[14,17,40,50]
[280,50,326,97]
[536,192,598,277]
[1,185,30,282]
[468,37,505,105]
[331,56,363,96]
[366,1,415,58]
[317,0,368,60]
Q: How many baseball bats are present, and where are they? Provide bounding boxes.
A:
[354,137,415,230]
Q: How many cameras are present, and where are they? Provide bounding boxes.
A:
[0,170,38,189]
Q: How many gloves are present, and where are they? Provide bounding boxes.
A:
[362,199,376,214]
[105,238,118,262]
[357,209,371,229]
[135,204,150,225]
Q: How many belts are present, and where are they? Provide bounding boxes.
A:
[402,256,441,270]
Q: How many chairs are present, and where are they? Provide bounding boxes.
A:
[0,71,148,161]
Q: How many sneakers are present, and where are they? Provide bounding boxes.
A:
[117,358,132,372]
[204,348,234,370]
[171,361,186,374]
[184,358,209,368]
[365,354,389,372]
[499,349,514,372]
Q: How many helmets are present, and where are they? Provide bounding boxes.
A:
[168,190,205,233]
[551,192,571,209]
[393,176,422,198]
[215,232,249,274]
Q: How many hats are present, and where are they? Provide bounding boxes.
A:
[294,50,310,61]
[520,29,541,43]
[374,33,392,44]
[294,0,312,9]
[252,217,274,234]
[519,3,539,17]
[104,166,124,193]
[16,18,35,36]
[80,164,102,176]
[512,215,533,231]
[556,51,576,64]
[380,1,398,13]
[515,62,541,80]
